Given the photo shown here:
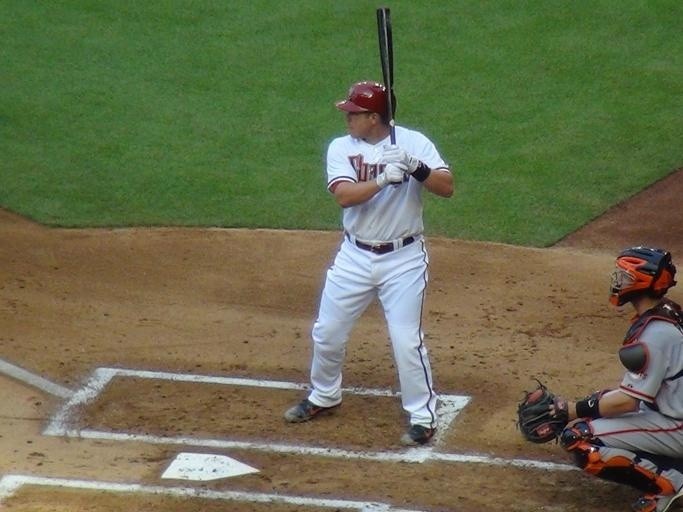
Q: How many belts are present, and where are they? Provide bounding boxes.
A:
[342,231,420,254]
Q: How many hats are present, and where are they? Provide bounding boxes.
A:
[333,81,396,119]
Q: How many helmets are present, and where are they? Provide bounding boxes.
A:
[606,244,676,307]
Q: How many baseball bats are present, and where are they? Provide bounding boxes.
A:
[376,8,402,186]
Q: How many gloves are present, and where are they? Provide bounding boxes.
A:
[378,145,421,174]
[377,163,407,188]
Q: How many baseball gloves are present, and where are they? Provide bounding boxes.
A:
[514,379,568,444]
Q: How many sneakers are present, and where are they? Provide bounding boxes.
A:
[635,485,683,512]
[400,424,436,446]
[284,400,342,424]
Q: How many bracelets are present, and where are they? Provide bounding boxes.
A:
[575,399,599,418]
[410,159,431,183]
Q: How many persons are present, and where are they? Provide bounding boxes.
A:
[515,244,683,512]
[280,77,456,448]
